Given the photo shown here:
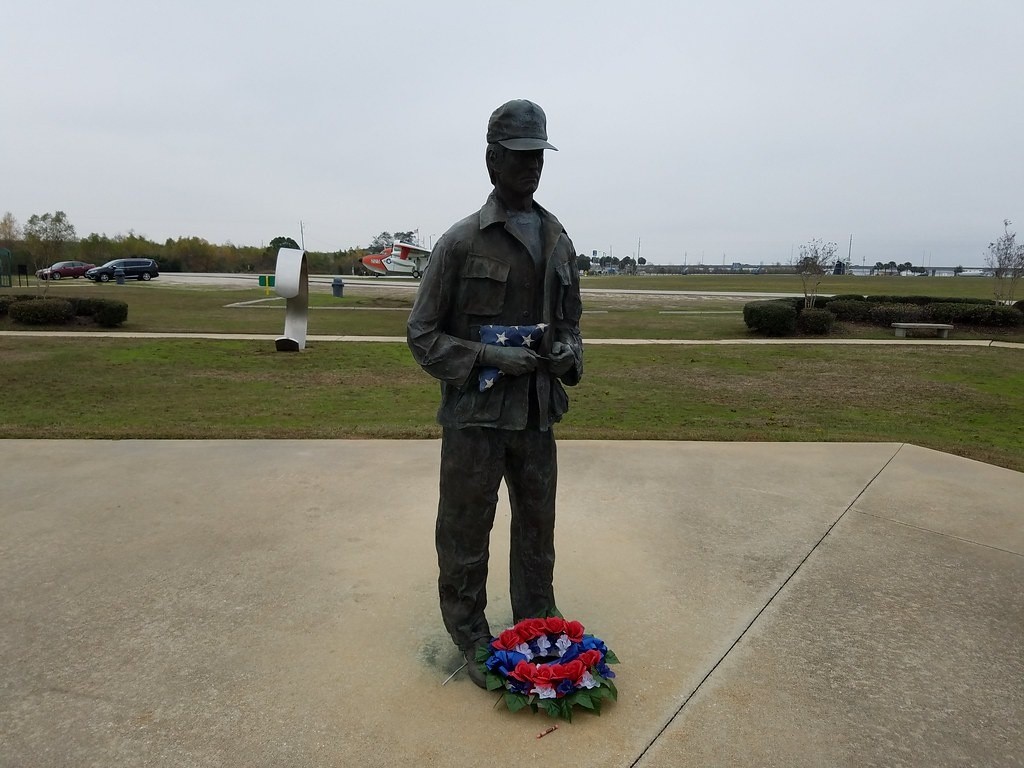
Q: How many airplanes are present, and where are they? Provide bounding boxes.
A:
[359,241,433,279]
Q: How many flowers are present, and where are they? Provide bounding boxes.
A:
[471,605,622,723]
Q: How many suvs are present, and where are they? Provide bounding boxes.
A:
[87,258,159,283]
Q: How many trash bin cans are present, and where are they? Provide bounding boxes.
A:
[331,278,344,297]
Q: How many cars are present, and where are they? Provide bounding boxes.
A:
[35,261,94,281]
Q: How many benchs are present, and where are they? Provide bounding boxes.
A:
[891,323,954,338]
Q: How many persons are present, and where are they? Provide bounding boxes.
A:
[406,99,584,691]
